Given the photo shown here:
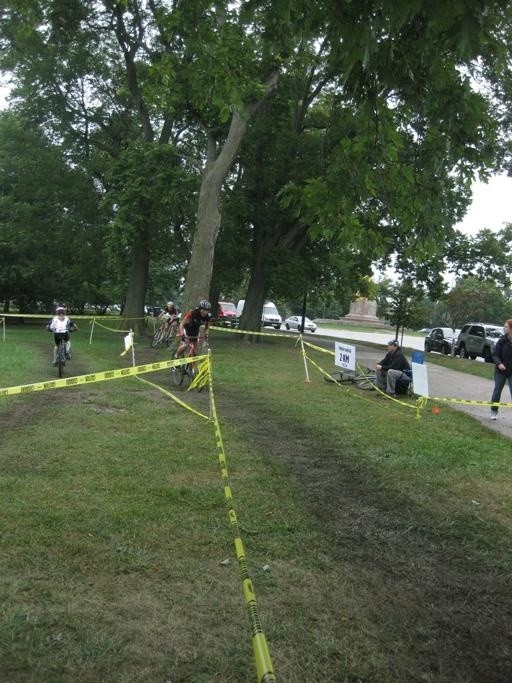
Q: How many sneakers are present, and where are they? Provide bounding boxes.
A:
[174,356,180,369]
[53,362,58,367]
[66,352,71,360]
[490,410,497,420]
[376,390,396,399]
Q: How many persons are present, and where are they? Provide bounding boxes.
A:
[373,339,413,400]
[155,300,178,338]
[489,317,512,420]
[44,305,78,368]
[174,298,212,376]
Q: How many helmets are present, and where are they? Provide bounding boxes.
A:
[167,301,175,306]
[56,307,65,311]
[198,300,211,308]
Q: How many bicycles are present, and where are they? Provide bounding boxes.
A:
[151,318,177,348]
[324,367,377,390]
[172,335,208,386]
[46,329,77,377]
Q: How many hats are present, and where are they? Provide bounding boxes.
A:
[388,340,399,345]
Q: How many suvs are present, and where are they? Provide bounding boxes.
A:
[458,323,505,363]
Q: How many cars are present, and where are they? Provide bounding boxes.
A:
[425,327,462,355]
[285,316,317,332]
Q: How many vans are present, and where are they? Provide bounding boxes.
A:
[237,300,282,329]
[217,302,237,318]
